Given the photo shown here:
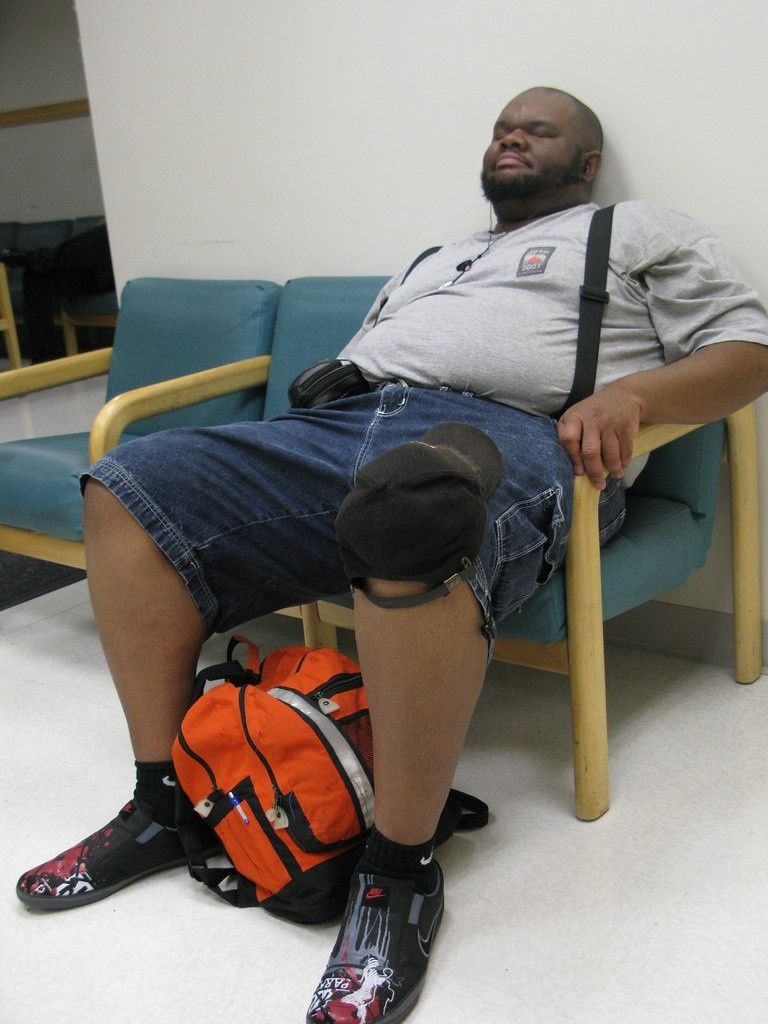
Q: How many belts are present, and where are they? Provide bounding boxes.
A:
[387,376,464,397]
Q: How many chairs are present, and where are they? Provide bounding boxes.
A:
[0,273,760,821]
[0,216,123,369]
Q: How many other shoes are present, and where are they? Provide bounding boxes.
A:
[302,858,451,1024]
[12,800,223,911]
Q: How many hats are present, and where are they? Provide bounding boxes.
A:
[333,422,506,605]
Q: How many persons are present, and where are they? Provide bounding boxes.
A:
[18,86,767,1023]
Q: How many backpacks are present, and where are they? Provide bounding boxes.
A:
[174,633,490,924]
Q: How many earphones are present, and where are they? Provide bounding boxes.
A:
[583,161,590,172]
